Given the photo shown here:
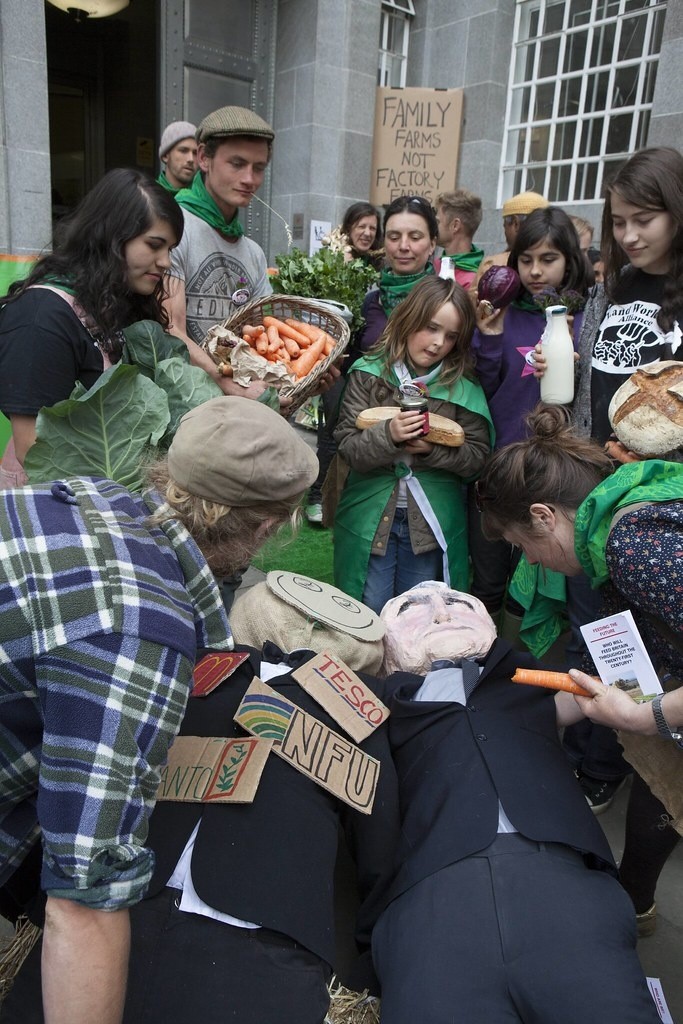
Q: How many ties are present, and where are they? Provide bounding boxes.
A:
[447,655,480,701]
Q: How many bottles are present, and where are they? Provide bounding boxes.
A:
[538,304,575,404]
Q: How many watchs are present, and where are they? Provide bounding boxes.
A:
[652,694,682,742]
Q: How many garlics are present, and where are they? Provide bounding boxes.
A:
[321,234,353,254]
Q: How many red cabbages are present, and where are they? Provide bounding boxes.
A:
[478,264,522,309]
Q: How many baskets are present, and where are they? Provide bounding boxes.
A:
[201,292,352,419]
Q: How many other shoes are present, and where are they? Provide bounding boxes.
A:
[636,901,657,938]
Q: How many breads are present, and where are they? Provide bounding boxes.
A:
[355,406,464,447]
[608,359,683,459]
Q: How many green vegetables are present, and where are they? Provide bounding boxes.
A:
[267,243,380,345]
[23,319,281,489]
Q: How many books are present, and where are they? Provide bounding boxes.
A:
[579,609,664,703]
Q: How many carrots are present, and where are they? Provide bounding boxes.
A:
[510,668,604,698]
[219,314,337,384]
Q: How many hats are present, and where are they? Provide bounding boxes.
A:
[158,121,197,165]
[167,394,321,509]
[501,191,550,219]
[193,105,275,147]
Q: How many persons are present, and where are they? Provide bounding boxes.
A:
[331,275,496,618]
[0,396,318,1024]
[128,579,663,1024]
[568,214,604,283]
[531,145,683,809]
[469,192,549,295]
[434,188,485,290]
[341,195,440,378]
[340,201,385,270]
[469,206,596,636]
[154,120,199,196]
[137,105,293,395]
[472,403,683,919]
[0,168,184,492]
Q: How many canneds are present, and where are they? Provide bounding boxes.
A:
[401,396,430,437]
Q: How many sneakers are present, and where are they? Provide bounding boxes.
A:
[305,504,323,522]
[577,772,628,815]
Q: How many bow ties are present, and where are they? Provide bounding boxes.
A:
[261,640,318,668]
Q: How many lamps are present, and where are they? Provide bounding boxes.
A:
[47,0,129,23]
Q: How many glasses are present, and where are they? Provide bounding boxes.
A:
[388,195,430,208]
